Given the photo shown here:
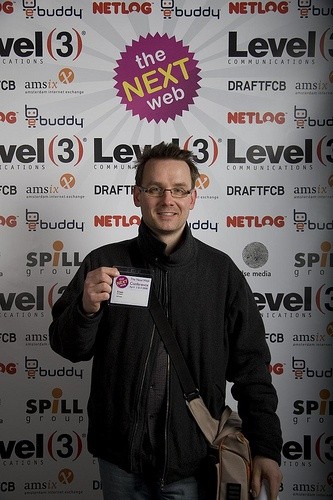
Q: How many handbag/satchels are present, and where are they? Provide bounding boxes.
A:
[188,390,251,499]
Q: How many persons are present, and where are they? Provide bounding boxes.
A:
[48,143,283,500]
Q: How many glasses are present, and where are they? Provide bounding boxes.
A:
[138,185,194,198]
